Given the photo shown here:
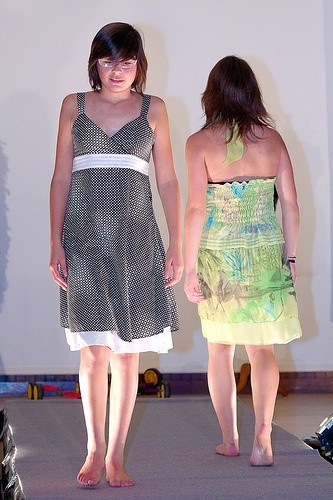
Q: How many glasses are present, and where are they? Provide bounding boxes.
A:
[96,57,139,70]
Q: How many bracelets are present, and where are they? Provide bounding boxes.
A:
[287,256,296,264]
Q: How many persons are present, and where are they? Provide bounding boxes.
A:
[46,21,184,488]
[183,52,303,465]
